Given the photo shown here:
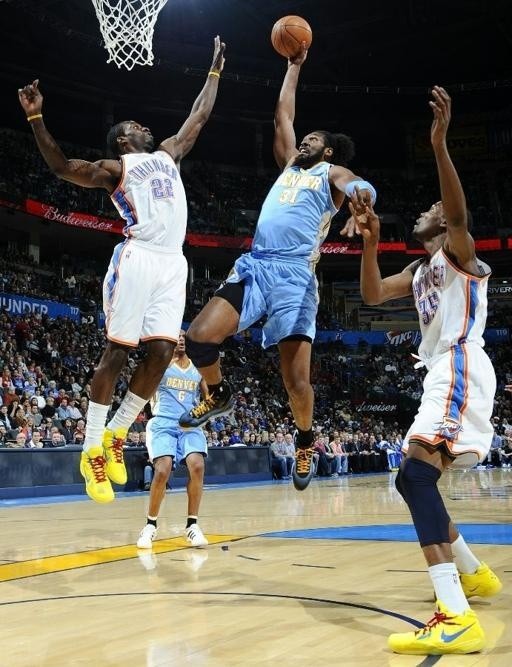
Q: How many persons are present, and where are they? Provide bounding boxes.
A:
[134,327,213,549]
[1,130,154,489]
[479,300,511,470]
[179,39,378,492]
[15,35,228,504]
[184,278,219,324]
[205,304,425,479]
[180,159,258,238]
[329,155,499,241]
[348,85,504,654]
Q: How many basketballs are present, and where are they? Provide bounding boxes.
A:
[271,16,312,57]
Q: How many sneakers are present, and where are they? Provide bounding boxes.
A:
[79,447,115,504]
[178,383,236,430]
[102,426,127,485]
[388,601,486,655]
[434,560,501,601]
[183,523,209,547]
[290,429,316,490]
[136,523,160,550]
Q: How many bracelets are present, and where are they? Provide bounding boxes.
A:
[207,71,220,79]
[26,114,43,122]
